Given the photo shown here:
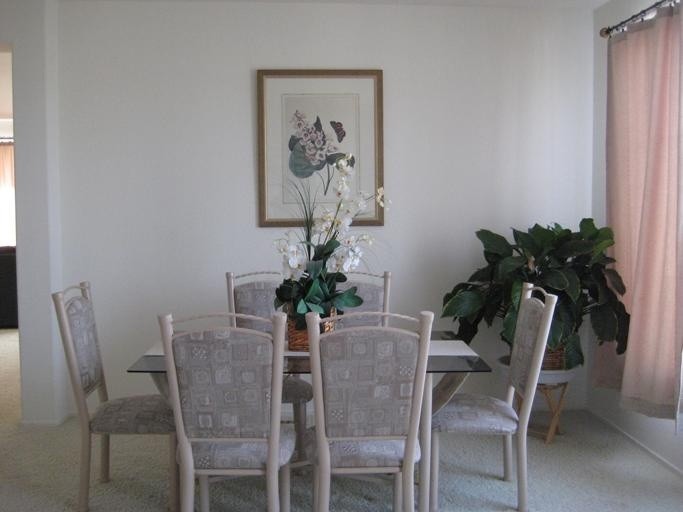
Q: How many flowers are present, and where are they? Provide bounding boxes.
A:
[285,108,358,198]
[273,151,393,326]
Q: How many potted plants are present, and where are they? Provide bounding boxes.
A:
[438,215,633,380]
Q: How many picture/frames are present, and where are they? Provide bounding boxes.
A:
[255,69,386,230]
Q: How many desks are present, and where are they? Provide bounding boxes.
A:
[127,330,493,512]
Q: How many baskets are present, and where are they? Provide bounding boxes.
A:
[285,303,336,351]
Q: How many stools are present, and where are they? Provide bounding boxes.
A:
[499,353,571,441]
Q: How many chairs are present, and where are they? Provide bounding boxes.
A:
[154,310,294,512]
[225,268,314,476]
[51,281,178,512]
[430,283,560,512]
[328,271,393,326]
[305,312,435,512]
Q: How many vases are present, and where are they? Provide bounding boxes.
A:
[288,310,337,350]
